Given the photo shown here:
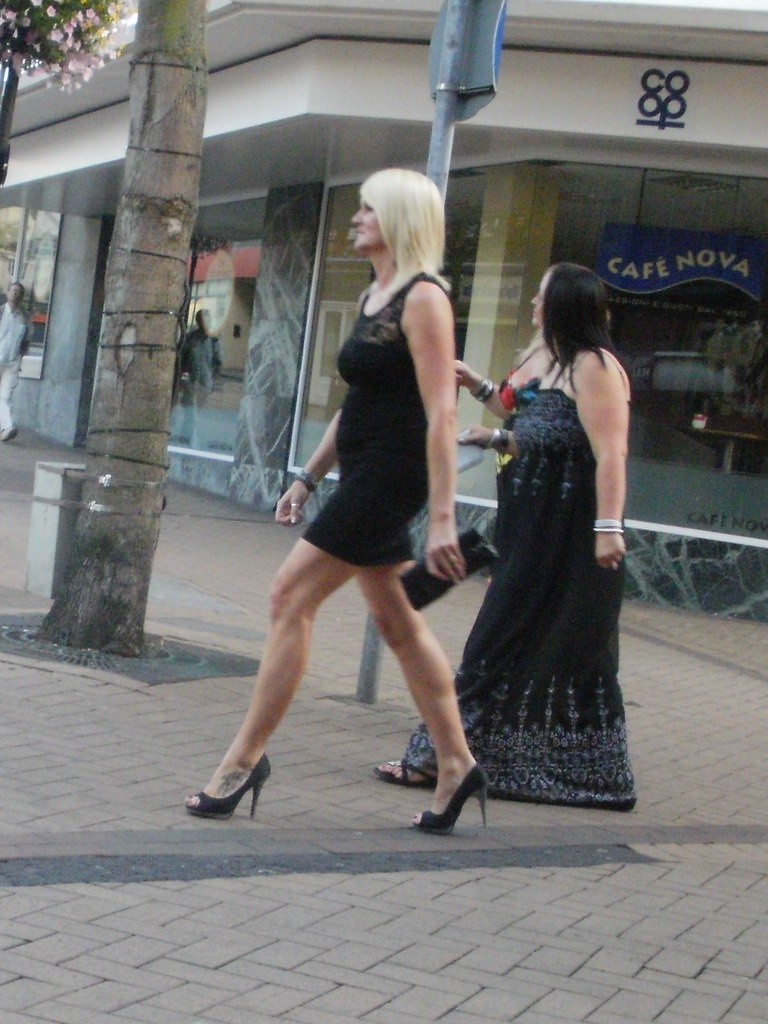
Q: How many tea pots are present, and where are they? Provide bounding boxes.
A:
[692,413,708,429]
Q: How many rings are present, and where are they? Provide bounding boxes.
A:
[291,503,299,507]
[448,555,455,562]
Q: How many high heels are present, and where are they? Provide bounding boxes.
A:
[185,752,272,821]
[411,761,488,836]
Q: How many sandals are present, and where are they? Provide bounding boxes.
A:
[373,758,440,788]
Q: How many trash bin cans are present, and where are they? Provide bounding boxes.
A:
[26,460,86,597]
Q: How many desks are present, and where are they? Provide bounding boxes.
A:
[697,427,761,474]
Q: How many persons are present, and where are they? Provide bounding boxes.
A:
[375,260,636,811]
[178,311,221,449]
[185,169,490,836]
[0,282,35,442]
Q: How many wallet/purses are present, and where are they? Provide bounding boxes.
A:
[398,527,499,611]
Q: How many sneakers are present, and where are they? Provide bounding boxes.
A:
[0,427,19,441]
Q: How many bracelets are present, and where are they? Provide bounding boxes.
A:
[592,527,623,534]
[472,376,493,403]
[595,520,621,528]
[294,469,317,492]
[490,429,508,455]
